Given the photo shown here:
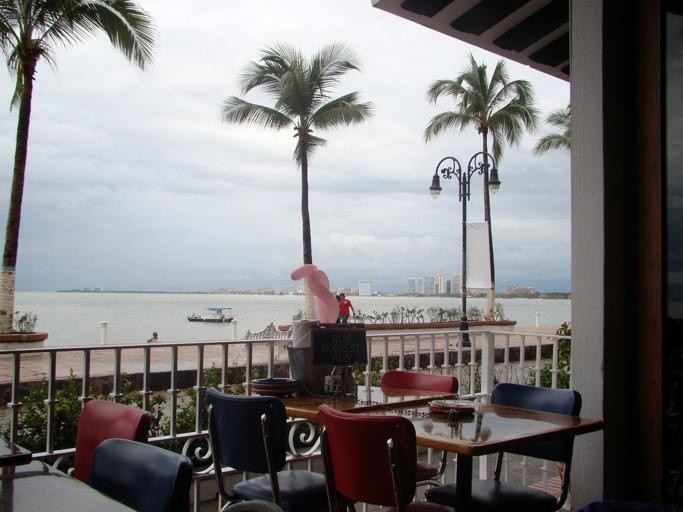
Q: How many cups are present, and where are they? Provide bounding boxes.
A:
[325,375,342,395]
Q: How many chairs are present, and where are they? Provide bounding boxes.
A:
[204,386,330,512]
[314,402,452,512]
[379,369,461,500]
[89,438,193,511]
[423,382,584,510]
[66,398,153,486]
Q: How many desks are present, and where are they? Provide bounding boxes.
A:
[251,385,458,426]
[356,400,606,509]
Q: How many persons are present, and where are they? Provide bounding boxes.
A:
[147,331,161,343]
[339,293,355,324]
[335,294,341,324]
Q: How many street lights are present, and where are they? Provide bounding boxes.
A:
[429,152,502,347]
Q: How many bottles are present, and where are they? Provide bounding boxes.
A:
[344,365,355,395]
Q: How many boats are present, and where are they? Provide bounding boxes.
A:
[186,304,234,322]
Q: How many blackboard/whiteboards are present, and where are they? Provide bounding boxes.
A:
[311,323,367,368]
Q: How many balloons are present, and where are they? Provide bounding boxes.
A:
[290,264,339,323]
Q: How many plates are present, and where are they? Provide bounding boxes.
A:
[249,378,299,395]
